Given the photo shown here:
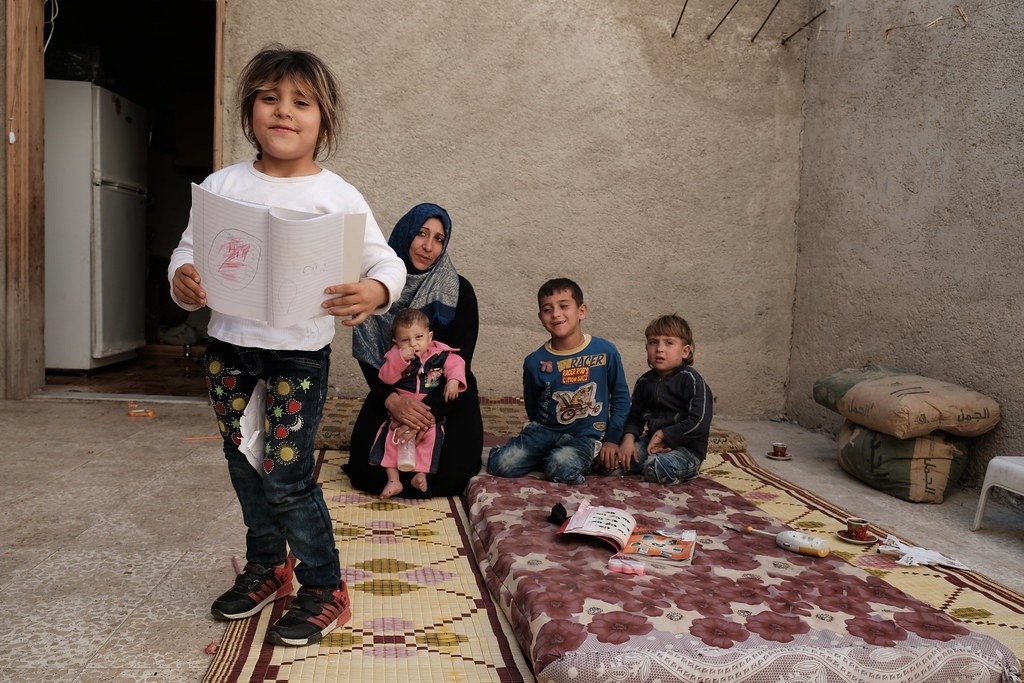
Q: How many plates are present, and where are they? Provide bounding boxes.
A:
[765,451,795,459]
[835,529,879,544]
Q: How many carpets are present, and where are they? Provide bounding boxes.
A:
[200,446,1024,683]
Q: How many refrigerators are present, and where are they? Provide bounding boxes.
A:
[41,78,156,371]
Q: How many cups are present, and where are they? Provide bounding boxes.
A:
[847,517,870,540]
[772,442,787,457]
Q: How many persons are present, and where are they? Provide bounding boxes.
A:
[592,314,713,481]
[339,203,483,499]
[486,278,629,481]
[168,44,407,646]
[376,308,468,501]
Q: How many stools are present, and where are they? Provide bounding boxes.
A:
[970,456,1024,532]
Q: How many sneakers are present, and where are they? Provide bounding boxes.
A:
[211,558,296,620]
[266,580,351,646]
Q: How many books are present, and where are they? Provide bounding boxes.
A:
[555,504,698,567]
[179,176,368,332]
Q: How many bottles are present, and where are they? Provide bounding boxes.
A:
[391,427,418,471]
